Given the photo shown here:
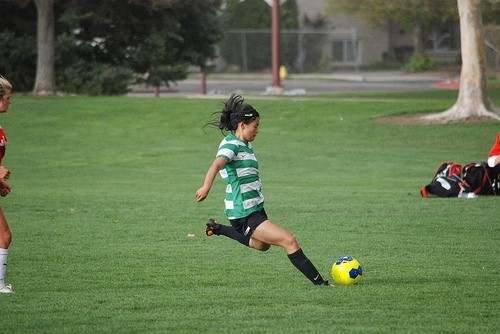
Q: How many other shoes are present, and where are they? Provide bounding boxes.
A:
[0,284,15,293]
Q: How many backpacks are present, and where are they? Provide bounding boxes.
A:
[420,162,462,197]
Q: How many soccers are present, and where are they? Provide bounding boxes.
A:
[331,256,363,286]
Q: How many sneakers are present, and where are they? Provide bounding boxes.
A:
[206,218,217,236]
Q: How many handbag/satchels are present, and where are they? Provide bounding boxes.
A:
[463,161,500,196]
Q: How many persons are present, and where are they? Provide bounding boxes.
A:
[0,76,19,293]
[194,92,333,288]
